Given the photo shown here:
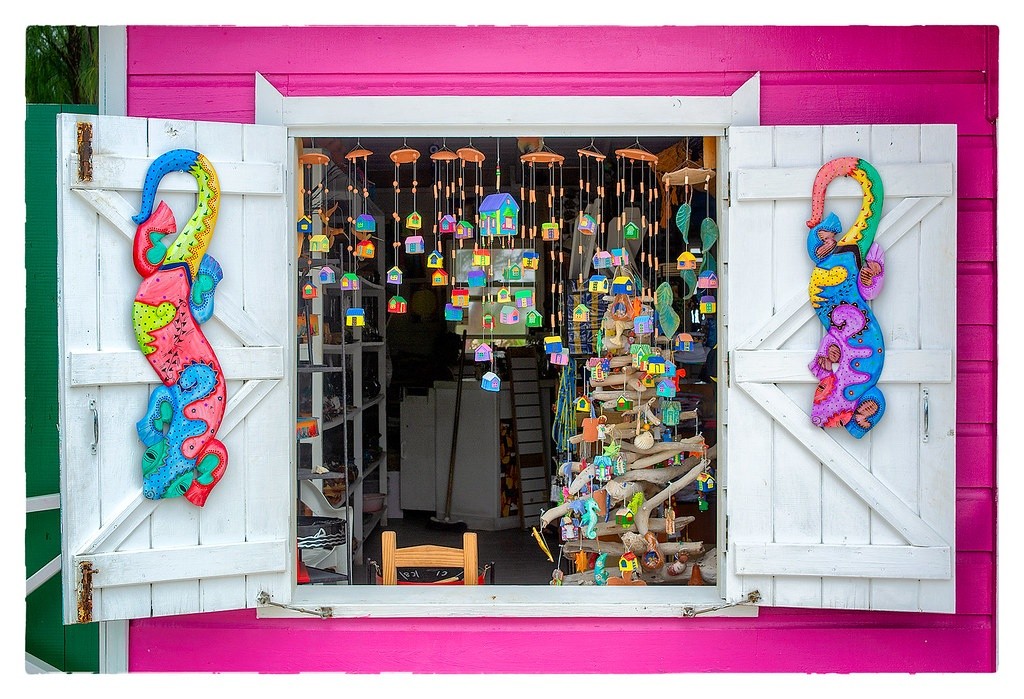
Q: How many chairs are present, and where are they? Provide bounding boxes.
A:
[366,531,494,586]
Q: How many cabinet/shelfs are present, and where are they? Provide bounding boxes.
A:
[296,147,388,588]
